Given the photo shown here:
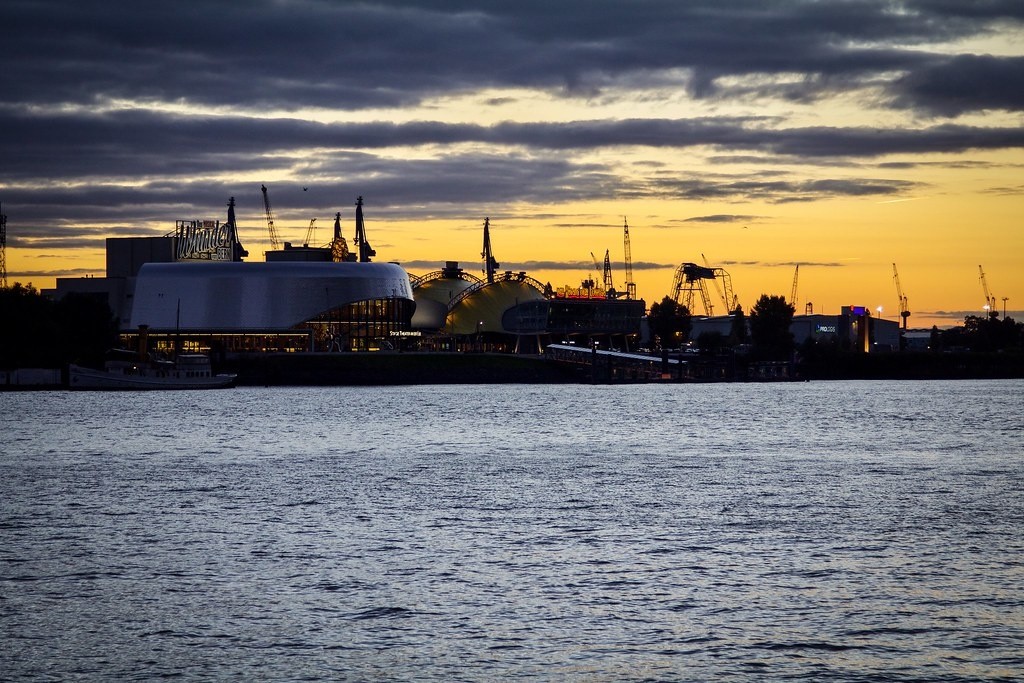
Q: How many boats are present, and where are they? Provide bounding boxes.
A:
[68,298,238,391]
